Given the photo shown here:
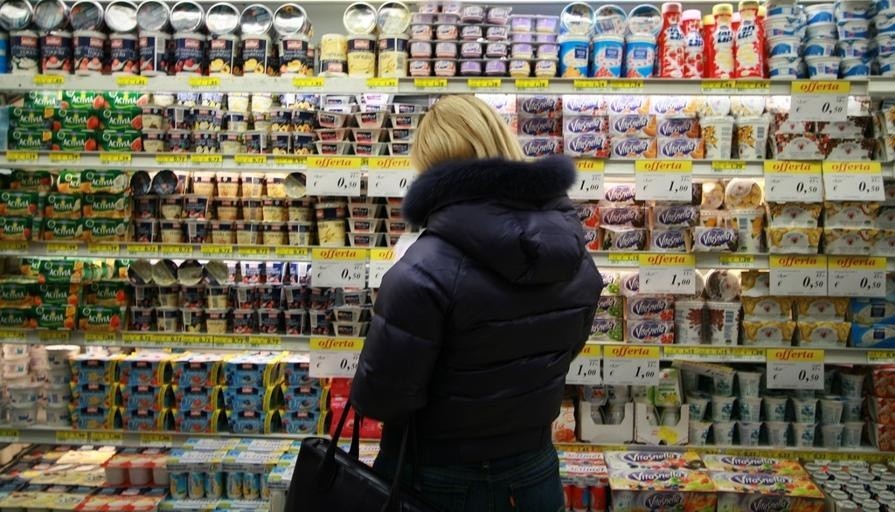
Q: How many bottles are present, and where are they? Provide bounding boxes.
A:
[659,1,763,80]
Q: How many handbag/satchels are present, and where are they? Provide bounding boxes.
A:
[285,437,398,512]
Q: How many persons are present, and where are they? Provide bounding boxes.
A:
[345,88,605,512]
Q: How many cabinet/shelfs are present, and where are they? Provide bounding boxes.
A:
[1,71,893,471]
